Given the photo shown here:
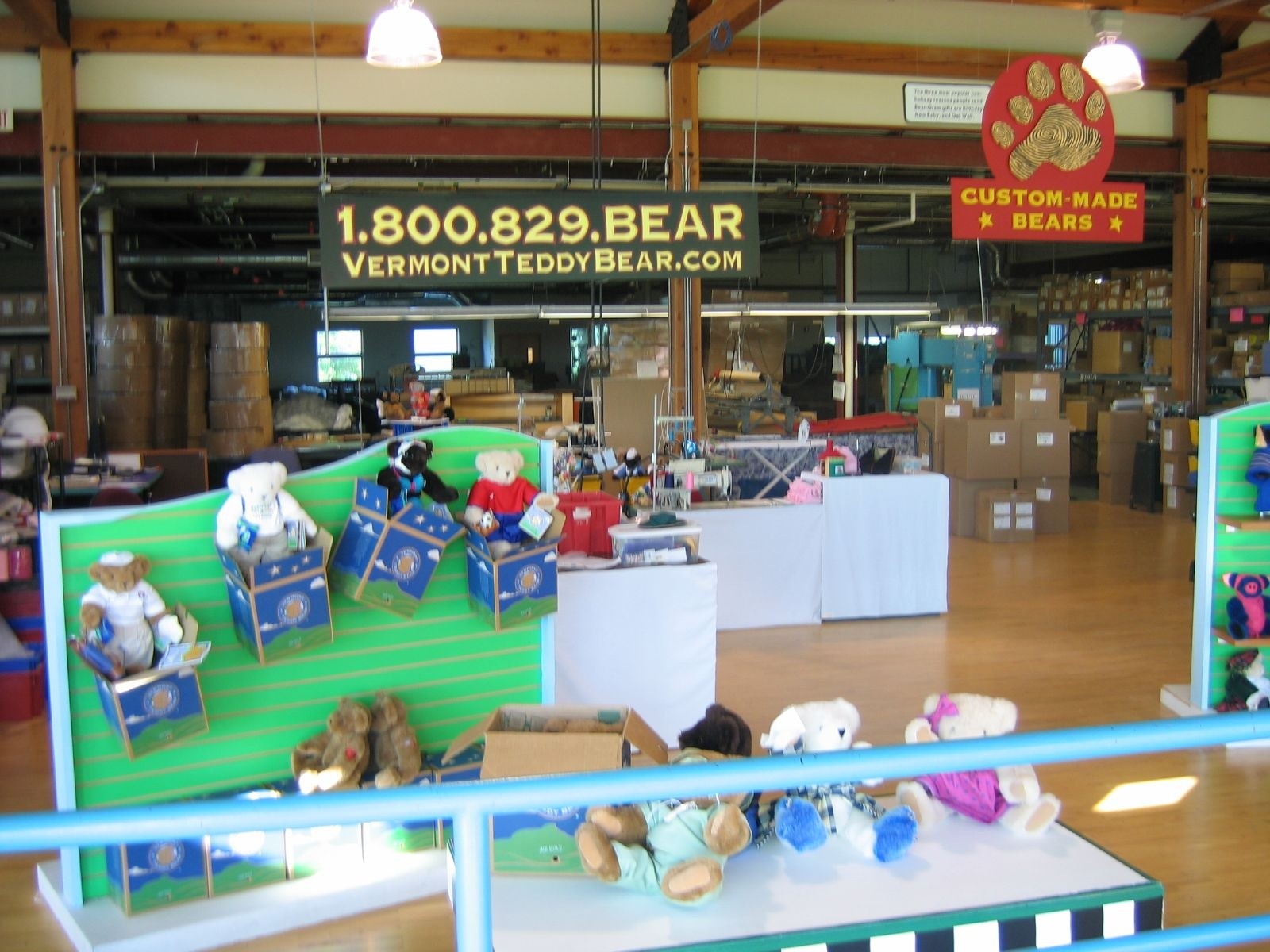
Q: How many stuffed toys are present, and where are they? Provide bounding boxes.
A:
[376,377,446,420]
[1221,572,1270,640]
[463,450,559,560]
[376,440,459,518]
[611,448,645,480]
[79,550,169,680]
[679,703,765,840]
[896,691,1061,842]
[290,697,371,792]
[215,461,318,578]
[369,690,421,790]
[763,695,918,865]
[1218,647,1270,712]
[573,747,754,907]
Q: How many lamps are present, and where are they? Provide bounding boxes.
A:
[321,300,943,323]
[1081,6,1148,97]
[364,0,444,70]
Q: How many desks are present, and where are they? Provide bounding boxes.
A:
[442,796,1166,952]
[804,471,951,619]
[292,438,367,466]
[48,467,165,503]
[630,498,824,629]
[551,564,719,745]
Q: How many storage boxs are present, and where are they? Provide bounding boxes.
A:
[916,262,1270,544]
[104,708,672,919]
[327,479,467,619]
[212,525,337,666]
[555,471,701,566]
[68,604,209,759]
[458,512,568,634]
[0,282,94,430]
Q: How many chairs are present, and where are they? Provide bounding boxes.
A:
[90,485,145,506]
[250,444,303,475]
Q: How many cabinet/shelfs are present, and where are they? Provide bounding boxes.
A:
[0,323,94,389]
[1158,398,1270,749]
[1035,302,1269,397]
[0,437,65,722]
